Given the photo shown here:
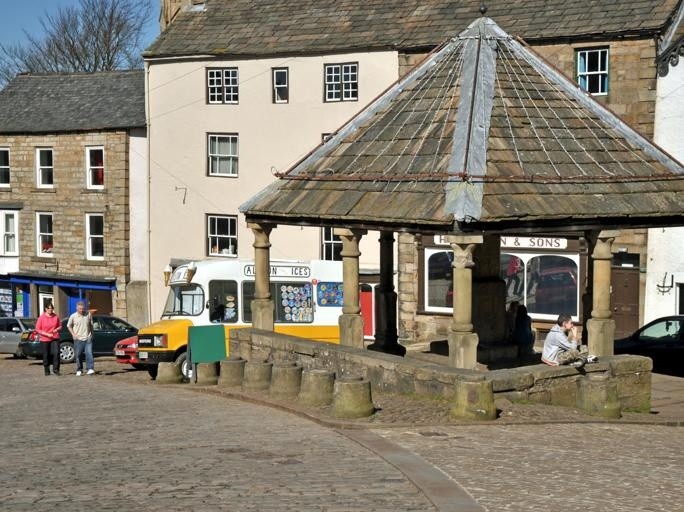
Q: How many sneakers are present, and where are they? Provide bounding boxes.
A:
[570,362,583,368]
[586,355,598,364]
[45,369,94,376]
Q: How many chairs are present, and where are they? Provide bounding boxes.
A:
[93,322,100,330]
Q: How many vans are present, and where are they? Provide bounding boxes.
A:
[138,258,343,383]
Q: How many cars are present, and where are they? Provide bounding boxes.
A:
[535,267,577,312]
[614,316,683,377]
[0,315,149,370]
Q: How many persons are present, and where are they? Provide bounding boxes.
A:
[541,314,598,369]
[515,304,536,354]
[67,301,95,376]
[504,301,528,357]
[527,255,541,293]
[504,256,520,296]
[35,303,63,376]
